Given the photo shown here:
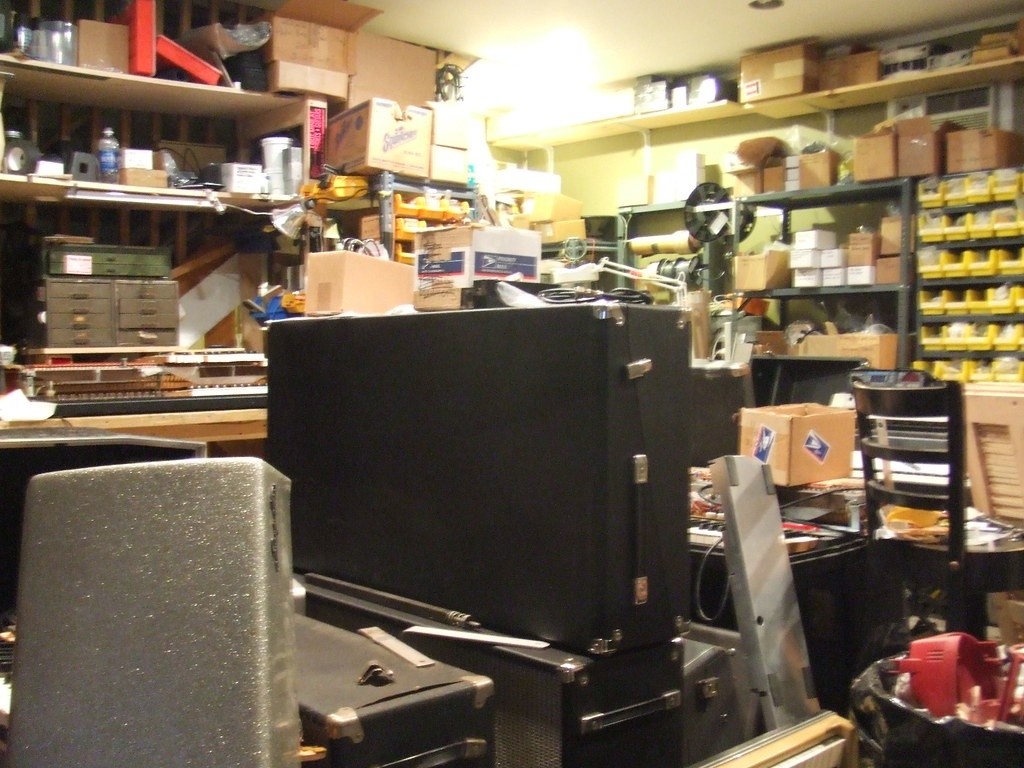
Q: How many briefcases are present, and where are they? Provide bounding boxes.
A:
[261,301,756,768]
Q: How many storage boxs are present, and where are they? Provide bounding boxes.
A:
[785,180,800,191]
[786,156,799,169]
[77,0,469,192]
[818,47,883,92]
[680,152,706,200]
[896,116,967,177]
[763,156,787,193]
[740,43,823,103]
[735,250,793,289]
[725,165,763,197]
[947,129,1024,174]
[790,215,915,290]
[303,195,587,317]
[619,175,655,209]
[756,322,898,370]
[972,20,1024,65]
[799,150,840,190]
[787,168,799,181]
[734,402,856,488]
[853,123,898,181]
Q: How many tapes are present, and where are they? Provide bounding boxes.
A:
[4,140,35,175]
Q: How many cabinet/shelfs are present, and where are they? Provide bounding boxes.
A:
[917,200,1024,382]
[618,178,917,405]
[31,243,178,348]
[0,52,381,212]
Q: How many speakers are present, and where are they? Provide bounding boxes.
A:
[5,305,757,768]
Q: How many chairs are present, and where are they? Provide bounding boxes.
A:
[853,379,1024,670]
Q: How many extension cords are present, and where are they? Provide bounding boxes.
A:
[380,173,394,233]
